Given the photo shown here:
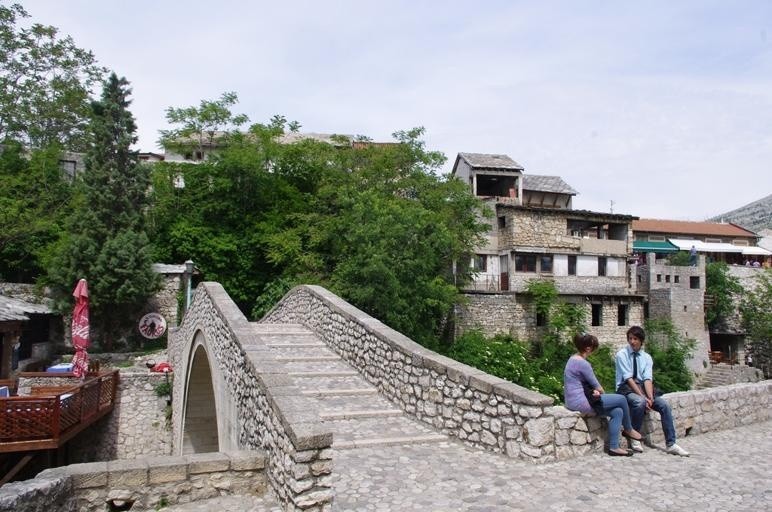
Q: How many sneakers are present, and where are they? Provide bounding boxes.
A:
[665,444,690,457]
[629,438,645,452]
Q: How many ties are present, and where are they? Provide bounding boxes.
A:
[631,352,637,379]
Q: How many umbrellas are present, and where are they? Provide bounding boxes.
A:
[70,278,91,384]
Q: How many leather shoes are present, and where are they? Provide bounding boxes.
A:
[622,430,645,441]
[608,449,634,457]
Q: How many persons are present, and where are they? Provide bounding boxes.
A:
[614,325,690,457]
[563,333,647,457]
